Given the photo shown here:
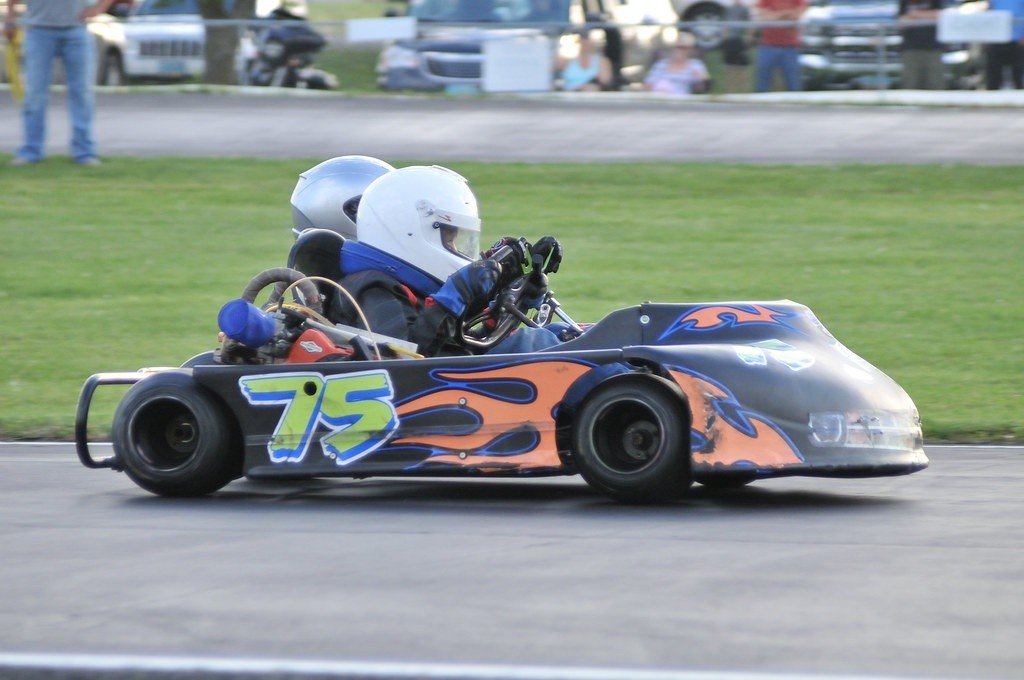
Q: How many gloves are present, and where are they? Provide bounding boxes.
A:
[528,235,564,273]
[481,235,533,276]
[429,258,504,321]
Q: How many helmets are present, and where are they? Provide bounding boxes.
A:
[287,152,398,248]
[354,163,486,282]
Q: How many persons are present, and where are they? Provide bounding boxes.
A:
[7,1,1023,166]
[290,155,563,353]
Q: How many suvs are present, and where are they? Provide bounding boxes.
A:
[798,0,905,90]
[375,0,756,91]
[84,1,256,83]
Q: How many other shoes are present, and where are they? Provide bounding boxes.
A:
[9,154,44,167]
[79,152,105,169]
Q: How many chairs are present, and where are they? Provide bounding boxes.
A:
[287,227,347,325]
